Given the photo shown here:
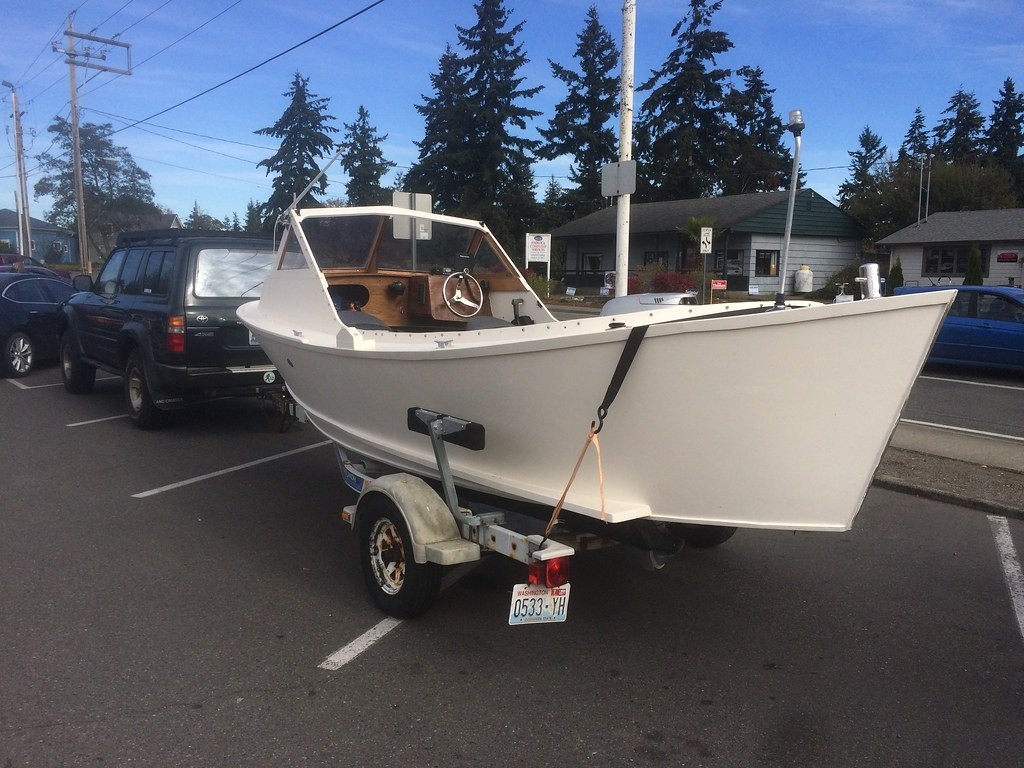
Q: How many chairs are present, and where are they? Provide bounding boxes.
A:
[952,299,964,317]
[986,297,1003,320]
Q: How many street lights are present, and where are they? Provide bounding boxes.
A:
[762,110,806,312]
[2,79,33,258]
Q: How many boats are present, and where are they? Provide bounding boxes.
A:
[236,149,961,534]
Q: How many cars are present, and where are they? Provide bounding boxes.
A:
[891,284,1024,377]
[0,252,85,378]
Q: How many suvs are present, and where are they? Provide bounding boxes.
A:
[54,230,283,429]
